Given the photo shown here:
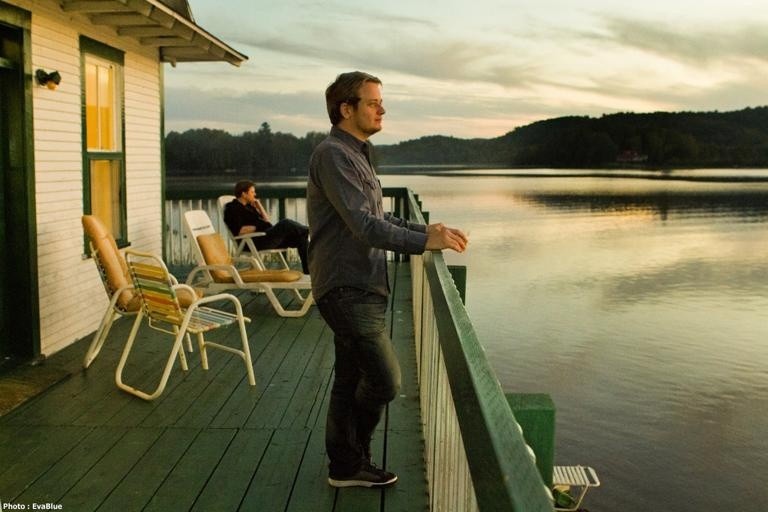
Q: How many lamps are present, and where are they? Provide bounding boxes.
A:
[36,68,61,91]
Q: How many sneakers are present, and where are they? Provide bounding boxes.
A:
[329,435,398,488]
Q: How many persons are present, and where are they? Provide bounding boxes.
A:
[223,183,310,276]
[304,68,470,489]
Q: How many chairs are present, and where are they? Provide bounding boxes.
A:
[215,195,290,270]
[116,249,257,401]
[81,215,201,372]
[185,210,318,318]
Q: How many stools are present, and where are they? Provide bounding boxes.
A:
[553,462,600,511]
